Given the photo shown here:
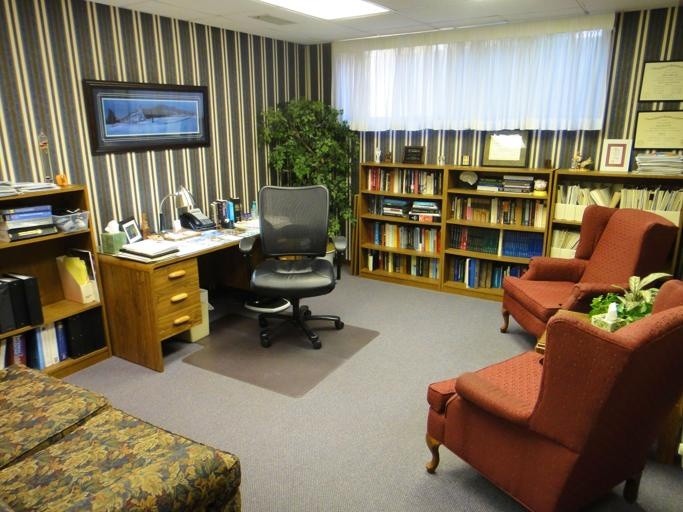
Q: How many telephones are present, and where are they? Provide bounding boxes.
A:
[180,208,216,231]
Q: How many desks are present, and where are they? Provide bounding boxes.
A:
[97,214,276,373]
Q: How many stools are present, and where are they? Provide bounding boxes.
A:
[1,407,242,507]
[0,361,107,471]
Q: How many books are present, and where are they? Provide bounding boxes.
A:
[476,174,534,193]
[0,180,61,199]
[365,249,440,280]
[632,153,683,174]
[368,221,442,253]
[451,195,547,228]
[0,321,68,372]
[367,166,442,195]
[449,224,500,255]
[210,197,243,225]
[114,238,180,263]
[69,247,96,280]
[502,229,544,258]
[1,204,58,244]
[552,226,581,250]
[449,256,529,289]
[556,182,683,213]
[0,271,45,336]
[368,194,442,223]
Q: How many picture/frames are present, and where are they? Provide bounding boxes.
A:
[120,217,144,244]
[81,78,212,153]
[599,136,633,173]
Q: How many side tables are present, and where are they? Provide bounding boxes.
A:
[534,308,613,353]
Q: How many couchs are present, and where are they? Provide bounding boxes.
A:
[497,205,679,352]
[426,281,683,507]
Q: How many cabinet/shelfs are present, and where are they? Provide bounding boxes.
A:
[546,163,611,261]
[0,181,112,386]
[360,158,559,301]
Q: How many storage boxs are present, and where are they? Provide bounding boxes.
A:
[51,208,90,234]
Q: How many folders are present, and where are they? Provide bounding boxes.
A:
[5,271,45,327]
[0,277,29,328]
[0,281,16,333]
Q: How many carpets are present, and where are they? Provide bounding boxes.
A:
[182,311,381,400]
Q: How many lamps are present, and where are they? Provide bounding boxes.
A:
[156,186,196,239]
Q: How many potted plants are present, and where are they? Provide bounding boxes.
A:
[258,98,358,276]
[483,130,528,168]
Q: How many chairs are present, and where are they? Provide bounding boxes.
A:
[250,184,347,348]
